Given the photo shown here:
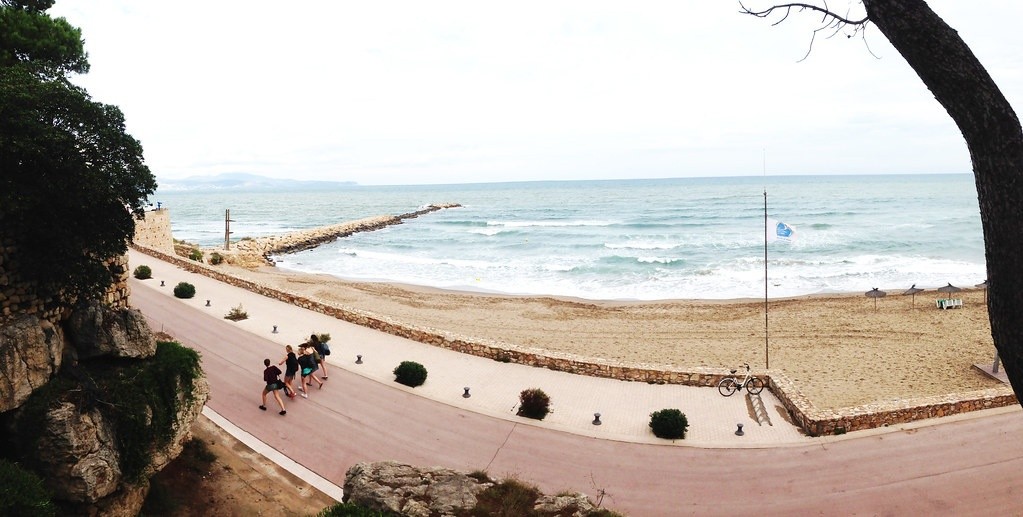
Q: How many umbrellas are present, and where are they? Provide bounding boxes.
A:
[866,280,988,311]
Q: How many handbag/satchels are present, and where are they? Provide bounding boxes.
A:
[312,347,321,363]
[277,380,283,390]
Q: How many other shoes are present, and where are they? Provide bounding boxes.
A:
[301,394,307,398]
[298,387,304,391]
[289,392,296,399]
[306,382,312,386]
[322,376,328,380]
[319,382,323,389]
[259,405,266,410]
[279,411,286,415]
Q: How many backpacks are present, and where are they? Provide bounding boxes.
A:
[289,354,298,371]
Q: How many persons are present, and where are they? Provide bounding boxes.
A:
[259,334,329,415]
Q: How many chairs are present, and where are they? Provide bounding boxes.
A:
[936,299,962,310]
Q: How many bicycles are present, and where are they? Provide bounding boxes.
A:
[718,363,764,397]
